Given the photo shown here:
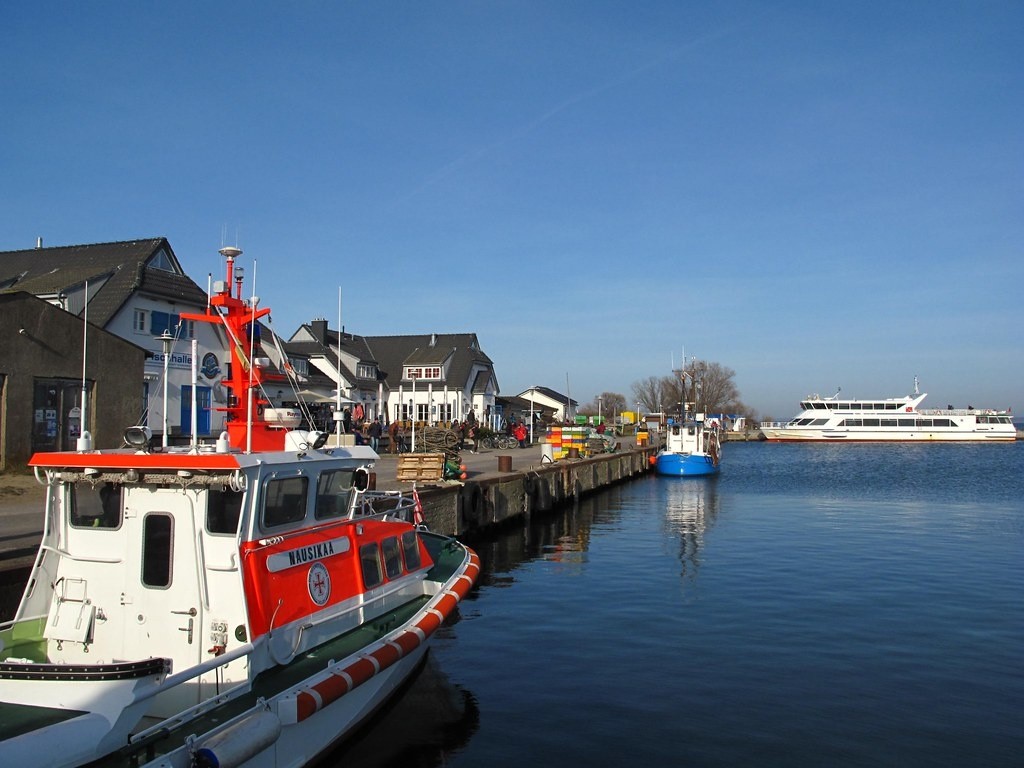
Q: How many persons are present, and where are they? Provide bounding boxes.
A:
[451,419,480,455]
[467,409,475,424]
[388,420,399,454]
[551,418,570,427]
[354,427,367,445]
[367,417,382,453]
[292,401,352,434]
[510,413,529,449]
[595,422,605,435]
[533,411,540,424]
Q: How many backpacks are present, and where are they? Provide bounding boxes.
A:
[468,426,475,437]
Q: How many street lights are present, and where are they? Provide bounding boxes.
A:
[528,386,539,443]
[598,397,603,425]
[154,329,178,447]
[636,402,640,423]
[409,370,420,453]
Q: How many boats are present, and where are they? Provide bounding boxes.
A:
[759,375,1017,442]
[655,345,722,476]
[1,246,481,768]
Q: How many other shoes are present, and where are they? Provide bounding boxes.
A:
[470,450,479,455]
[385,449,388,452]
